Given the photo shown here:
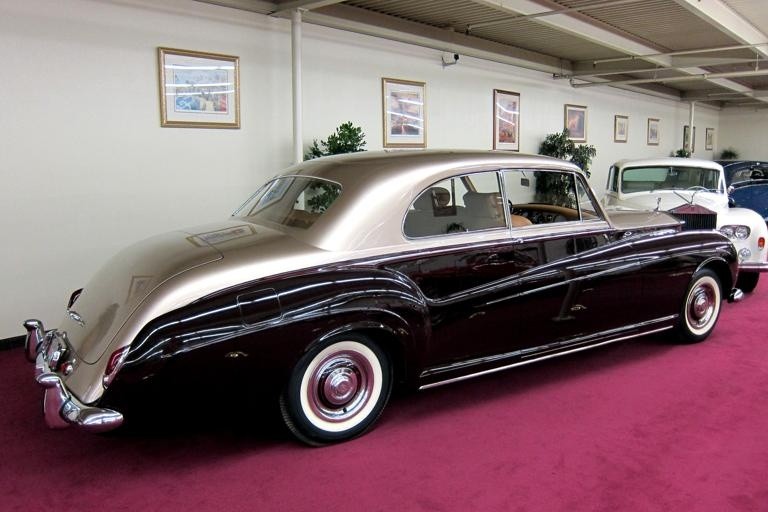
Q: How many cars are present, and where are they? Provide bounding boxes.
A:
[600,158,768,301]
[741,161,768,221]
[23,152,740,450]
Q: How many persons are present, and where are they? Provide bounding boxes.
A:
[173,69,228,113]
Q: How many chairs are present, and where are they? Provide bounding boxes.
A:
[408,186,465,236]
[462,190,529,232]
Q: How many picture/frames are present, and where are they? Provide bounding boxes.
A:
[380,74,428,151]
[705,126,716,153]
[682,125,697,153]
[492,86,523,153]
[561,102,589,144]
[646,115,659,146]
[158,46,244,133]
[612,114,630,145]
